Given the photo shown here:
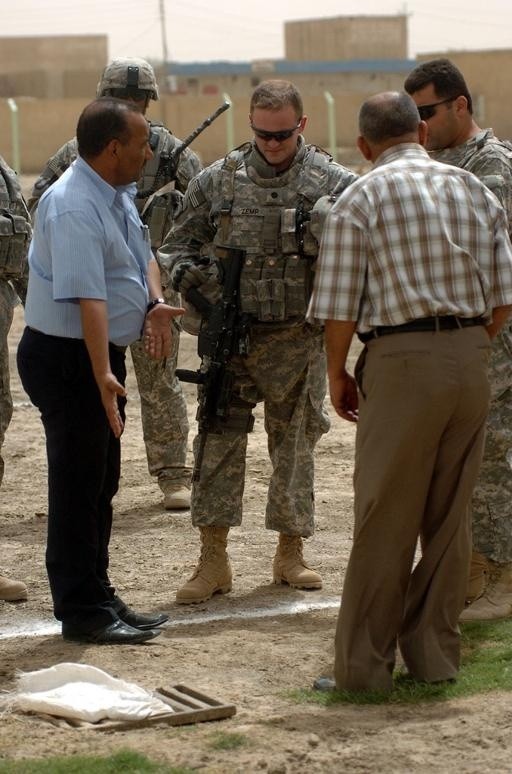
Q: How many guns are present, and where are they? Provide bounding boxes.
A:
[173,248,255,483]
[138,103,229,201]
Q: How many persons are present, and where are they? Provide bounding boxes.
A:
[1,149,28,601]
[32,57,204,510]
[408,56,512,619]
[304,91,512,705]
[17,98,169,643]
[158,79,359,603]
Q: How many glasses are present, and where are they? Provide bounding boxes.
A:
[416,96,458,121]
[250,119,304,142]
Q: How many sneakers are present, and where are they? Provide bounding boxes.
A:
[163,483,191,509]
[0,576,28,602]
[312,676,337,693]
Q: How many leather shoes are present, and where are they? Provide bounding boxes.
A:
[62,618,162,643]
[117,605,170,631]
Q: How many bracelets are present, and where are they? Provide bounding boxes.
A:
[146,299,165,312]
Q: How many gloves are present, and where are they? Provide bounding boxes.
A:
[168,259,206,294]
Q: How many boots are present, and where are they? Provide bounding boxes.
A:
[175,526,233,605]
[458,562,512,625]
[464,552,489,606]
[271,531,323,590]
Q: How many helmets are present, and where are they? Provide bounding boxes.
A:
[94,56,160,102]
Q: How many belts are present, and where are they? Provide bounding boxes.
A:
[356,314,487,344]
